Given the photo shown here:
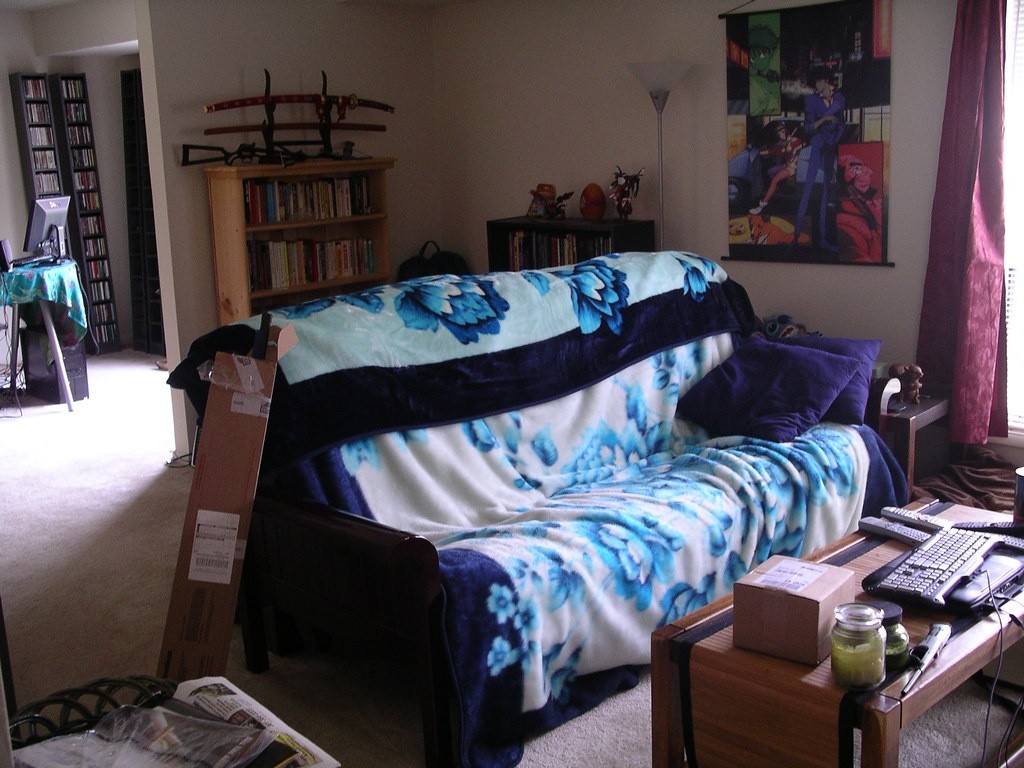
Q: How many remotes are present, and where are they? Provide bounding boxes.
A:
[881,507,948,530]
[1001,534,1024,551]
[858,516,931,546]
[950,521,1024,535]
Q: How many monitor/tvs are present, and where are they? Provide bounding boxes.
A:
[23,196,71,268]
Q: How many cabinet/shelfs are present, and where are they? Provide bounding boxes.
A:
[486,216,656,274]
[202,158,396,328]
[9,70,74,260]
[49,73,123,356]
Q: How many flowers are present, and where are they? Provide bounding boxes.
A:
[608,165,645,208]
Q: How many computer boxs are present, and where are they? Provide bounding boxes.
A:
[19,327,89,404]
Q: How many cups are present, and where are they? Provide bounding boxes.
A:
[1013,467,1024,520]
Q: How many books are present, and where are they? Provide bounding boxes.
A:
[24,77,58,196]
[244,175,382,226]
[508,229,615,272]
[247,234,378,292]
[61,79,119,344]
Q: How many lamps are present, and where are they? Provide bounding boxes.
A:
[625,60,700,250]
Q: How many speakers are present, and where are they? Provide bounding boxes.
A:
[0,239,13,273]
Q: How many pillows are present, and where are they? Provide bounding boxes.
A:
[674,335,862,442]
[768,339,881,426]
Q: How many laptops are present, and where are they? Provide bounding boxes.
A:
[863,548,1024,610]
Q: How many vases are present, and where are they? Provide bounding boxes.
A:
[614,189,633,219]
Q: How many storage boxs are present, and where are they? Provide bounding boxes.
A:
[733,555,854,667]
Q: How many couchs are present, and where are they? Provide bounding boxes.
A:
[167,248,911,768]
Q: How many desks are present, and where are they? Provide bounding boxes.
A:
[0,256,78,411]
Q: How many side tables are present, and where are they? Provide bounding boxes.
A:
[865,394,956,503]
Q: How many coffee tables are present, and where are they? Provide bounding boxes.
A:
[651,497,1024,768]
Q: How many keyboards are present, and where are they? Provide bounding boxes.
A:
[876,526,1004,606]
[12,255,53,267]
